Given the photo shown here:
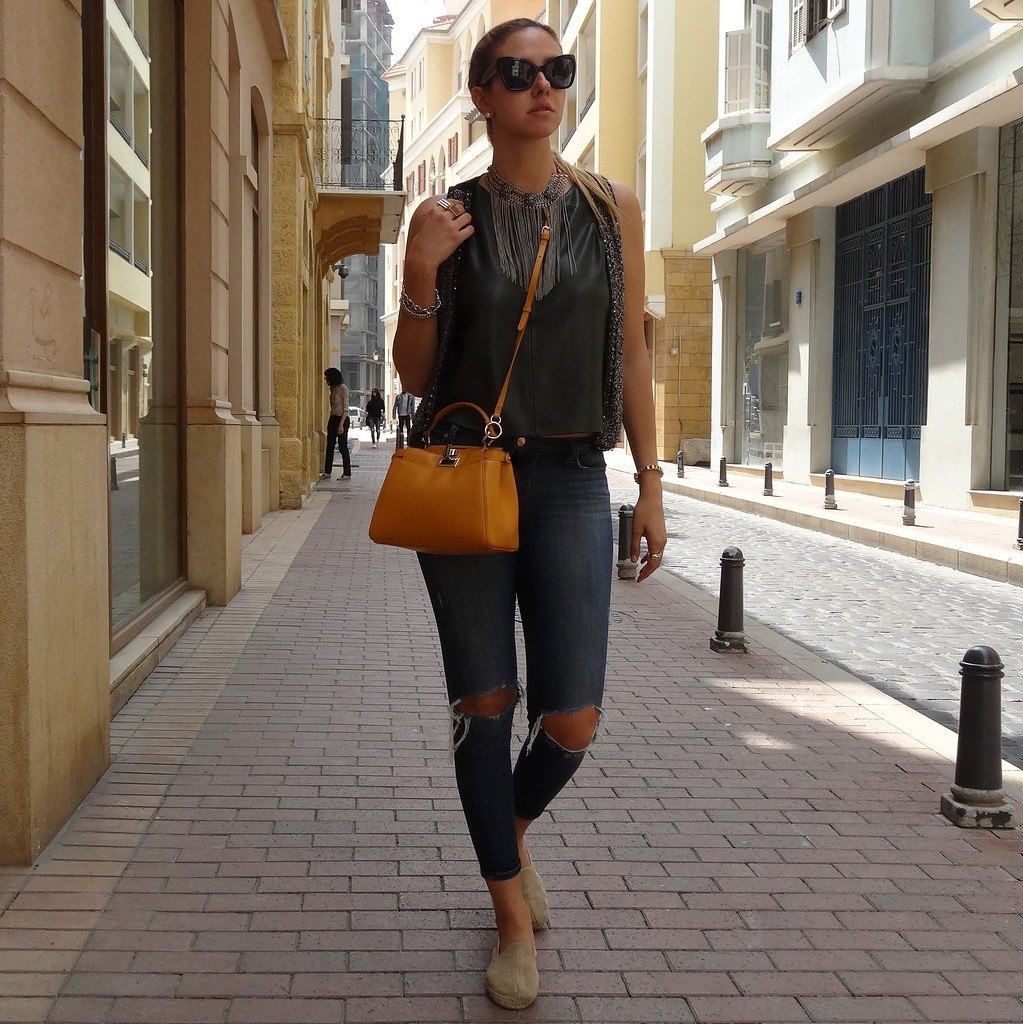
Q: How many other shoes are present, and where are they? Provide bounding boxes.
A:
[485,932,539,1009]
[336,475,352,480]
[319,474,332,480]
[519,837,549,930]
[373,443,376,447]
[377,440,379,445]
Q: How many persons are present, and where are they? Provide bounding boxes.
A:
[391,18,667,1007]
[393,386,415,445]
[321,368,351,481]
[363,388,385,448]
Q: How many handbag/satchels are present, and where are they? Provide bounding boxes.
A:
[369,445,519,553]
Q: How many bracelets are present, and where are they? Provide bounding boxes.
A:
[634,465,664,484]
[400,286,441,319]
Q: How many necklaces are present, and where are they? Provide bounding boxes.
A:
[486,165,577,302]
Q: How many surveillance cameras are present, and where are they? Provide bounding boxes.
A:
[339,267,350,279]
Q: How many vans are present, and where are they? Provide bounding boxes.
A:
[348,406,366,428]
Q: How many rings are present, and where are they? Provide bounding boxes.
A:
[437,199,451,210]
[647,553,662,559]
[448,206,463,216]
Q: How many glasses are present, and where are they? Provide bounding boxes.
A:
[482,54,576,91]
